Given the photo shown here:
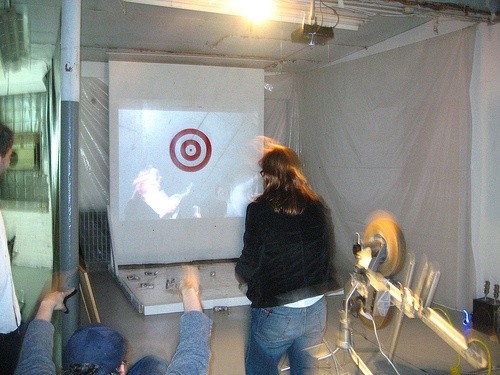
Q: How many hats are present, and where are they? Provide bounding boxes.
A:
[61,324,124,375]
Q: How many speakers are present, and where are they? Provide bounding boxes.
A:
[472,297,500,336]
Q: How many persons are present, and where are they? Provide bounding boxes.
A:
[134,168,192,221]
[235,147,330,375]
[0,124,22,375]
[15,279,212,375]
[228,135,274,217]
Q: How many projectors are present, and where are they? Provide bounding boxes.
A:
[292,23,334,45]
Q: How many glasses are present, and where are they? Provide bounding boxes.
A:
[260,170,264,177]
